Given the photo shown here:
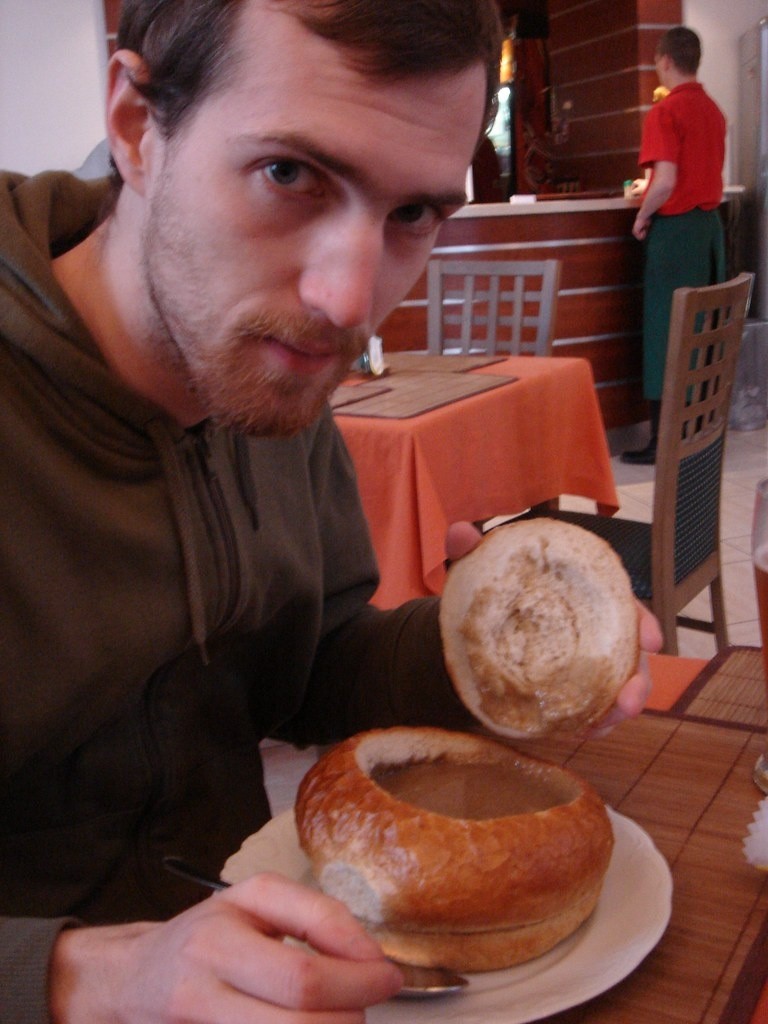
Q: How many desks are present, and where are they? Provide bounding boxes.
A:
[461,653,768,1023]
[334,353,620,610]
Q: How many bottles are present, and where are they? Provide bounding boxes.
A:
[624,179,633,199]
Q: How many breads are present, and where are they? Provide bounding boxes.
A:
[438,516,639,740]
[294,728,615,972]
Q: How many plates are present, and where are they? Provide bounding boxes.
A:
[280,804,674,1024]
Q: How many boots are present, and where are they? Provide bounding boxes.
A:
[622,402,660,464]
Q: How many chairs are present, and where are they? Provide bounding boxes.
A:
[430,273,756,655]
[427,258,563,533]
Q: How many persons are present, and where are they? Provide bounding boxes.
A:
[0,1,663,1024]
[621,27,727,464]
